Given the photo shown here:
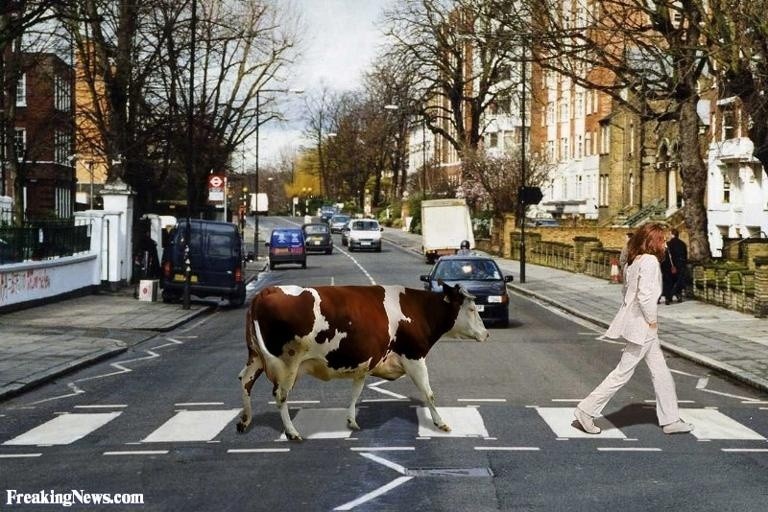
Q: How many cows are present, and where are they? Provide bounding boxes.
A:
[236,278,489,442]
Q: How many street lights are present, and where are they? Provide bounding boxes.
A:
[325,127,361,212]
[302,186,312,214]
[250,86,307,263]
[382,96,405,220]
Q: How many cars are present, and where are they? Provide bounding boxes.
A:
[418,250,512,327]
[533,218,560,228]
[299,222,334,254]
[327,214,349,233]
[339,219,349,244]
[318,208,334,222]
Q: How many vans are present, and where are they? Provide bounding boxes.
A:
[264,226,308,270]
[158,217,255,307]
[344,219,383,253]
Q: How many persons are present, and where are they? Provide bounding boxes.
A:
[620,232,635,295]
[669,228,687,303]
[658,243,677,305]
[457,241,470,254]
[574,222,696,434]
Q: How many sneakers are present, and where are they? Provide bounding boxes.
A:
[663,420,695,434]
[573,407,600,434]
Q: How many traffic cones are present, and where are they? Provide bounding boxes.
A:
[606,257,621,285]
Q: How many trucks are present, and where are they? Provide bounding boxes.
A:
[243,191,271,217]
[418,195,475,265]
